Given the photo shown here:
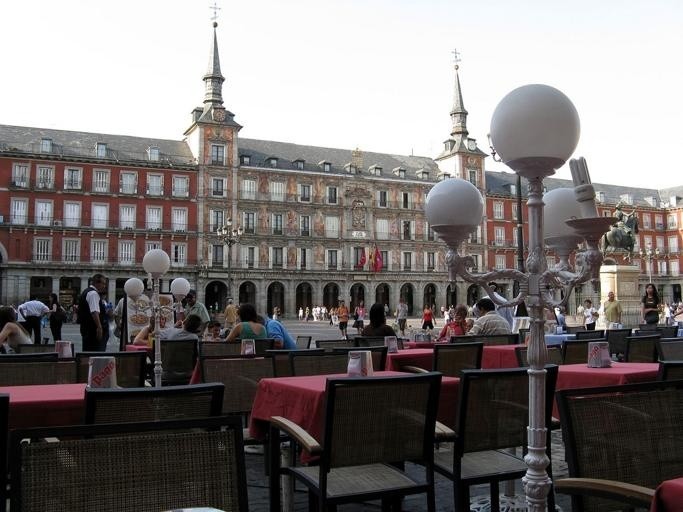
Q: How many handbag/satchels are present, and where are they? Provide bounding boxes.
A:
[645,313,658,324]
[353,312,359,320]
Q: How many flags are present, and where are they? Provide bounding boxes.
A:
[359,240,384,272]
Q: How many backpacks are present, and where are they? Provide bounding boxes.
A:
[59,306,67,323]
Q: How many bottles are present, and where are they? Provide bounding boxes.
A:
[425,326,432,341]
[407,324,413,338]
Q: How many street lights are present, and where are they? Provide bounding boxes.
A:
[122,246,193,388]
[215,217,243,312]
[415,79,614,506]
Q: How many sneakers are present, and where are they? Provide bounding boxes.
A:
[340,336,344,340]
[344,335,349,340]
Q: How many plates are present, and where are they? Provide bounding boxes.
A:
[128,296,172,337]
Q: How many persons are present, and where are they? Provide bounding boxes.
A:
[0,292,26,359]
[582,292,622,329]
[640,283,683,326]
[546,304,566,331]
[18,294,50,343]
[48,293,67,341]
[79,273,110,352]
[299,298,408,339]
[98,287,297,389]
[421,282,512,343]
